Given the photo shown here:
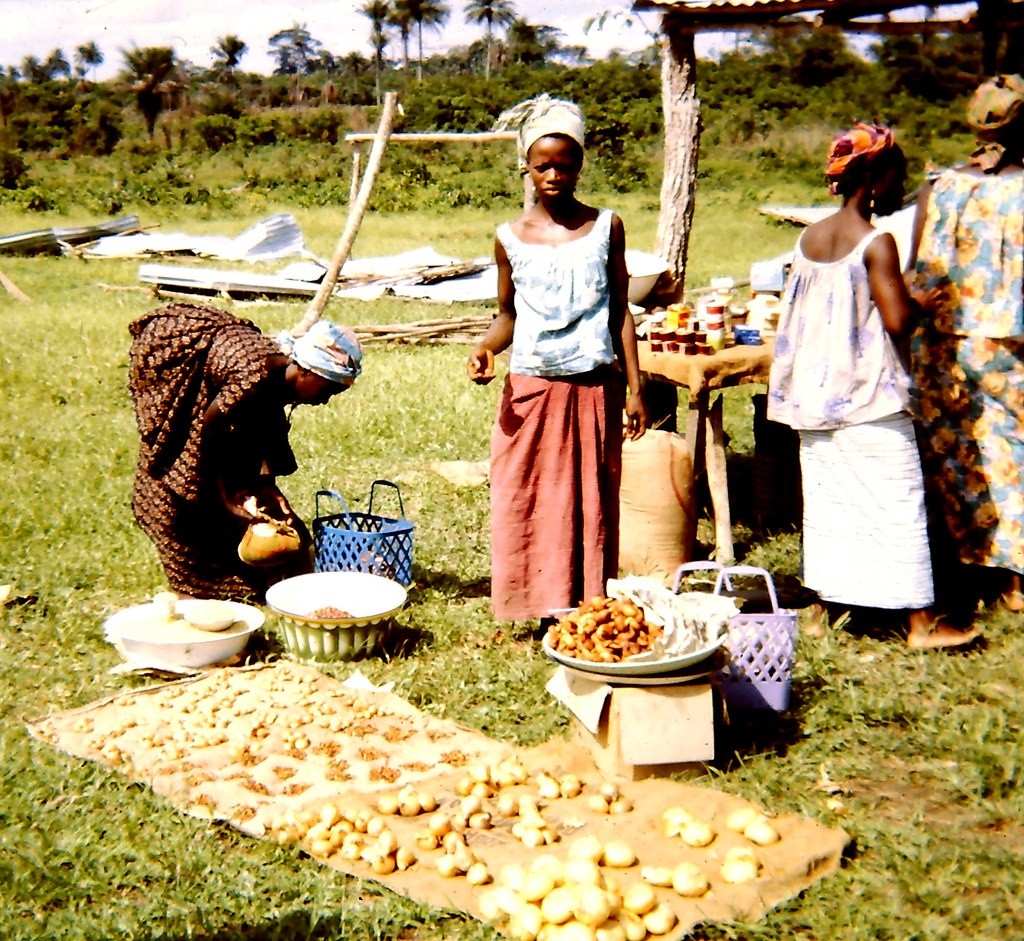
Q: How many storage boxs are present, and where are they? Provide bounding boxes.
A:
[544,667,729,780]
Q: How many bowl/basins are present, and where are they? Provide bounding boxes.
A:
[103,599,266,668]
[264,572,407,662]
[625,249,671,302]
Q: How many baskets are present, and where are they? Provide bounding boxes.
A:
[313,480,412,584]
[672,560,799,712]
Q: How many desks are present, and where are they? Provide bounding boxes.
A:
[633,336,777,564]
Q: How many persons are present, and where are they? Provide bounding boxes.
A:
[766,120,982,650]
[466,93,647,619]
[617,378,700,588]
[901,73,1024,613]
[128,304,363,608]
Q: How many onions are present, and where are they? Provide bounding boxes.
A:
[270,754,779,941]
[547,594,664,664]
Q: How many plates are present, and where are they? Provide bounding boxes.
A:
[542,625,729,676]
